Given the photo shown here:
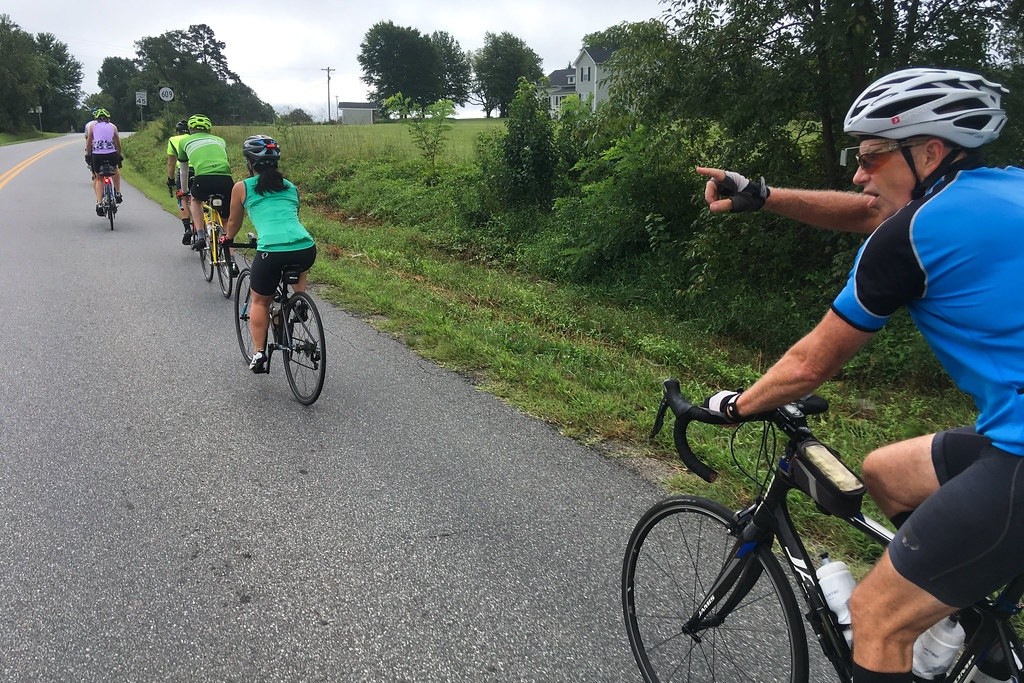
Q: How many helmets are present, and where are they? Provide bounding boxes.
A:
[242,135,281,161]
[843,68,1010,149]
[176,119,189,132]
[94,108,111,118]
[92,111,97,115]
[187,114,213,130]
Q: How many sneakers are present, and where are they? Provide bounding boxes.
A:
[116,196,122,202]
[96,205,103,215]
[191,239,206,250]
[293,299,307,319]
[231,262,239,274]
[182,229,192,244]
[250,351,268,370]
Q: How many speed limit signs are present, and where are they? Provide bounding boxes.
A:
[135,91,147,106]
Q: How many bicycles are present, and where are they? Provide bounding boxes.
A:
[168,176,240,298]
[84,146,118,231]
[619,379,1024,682]
[217,233,326,405]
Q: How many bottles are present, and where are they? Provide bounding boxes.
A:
[273,294,281,324]
[910,611,965,680]
[817,553,857,648]
[207,221,211,235]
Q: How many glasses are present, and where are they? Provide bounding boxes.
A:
[855,138,961,170]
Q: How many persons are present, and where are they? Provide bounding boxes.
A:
[84,107,124,216]
[177,114,240,276]
[167,119,213,245]
[697,68,1024,683]
[220,135,317,369]
